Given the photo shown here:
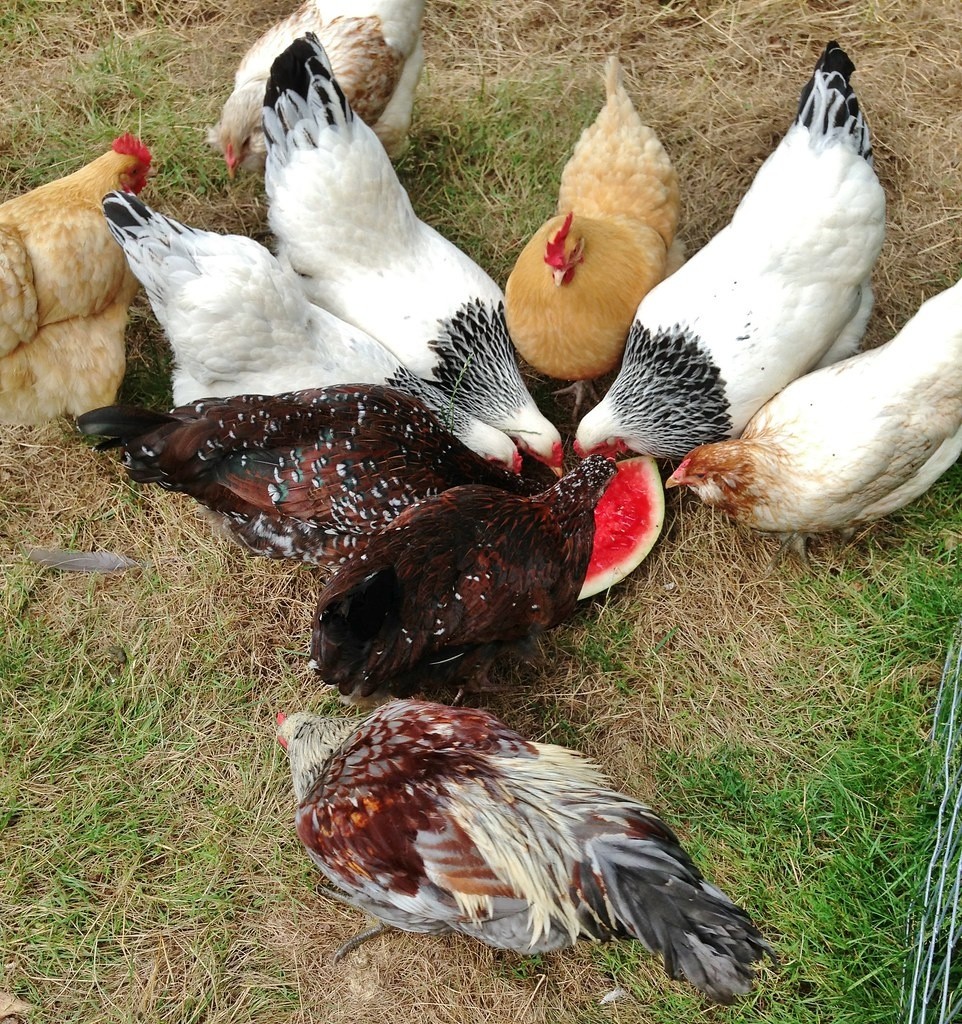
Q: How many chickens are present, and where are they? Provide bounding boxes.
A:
[0,2,962,698]
[276,697,780,1008]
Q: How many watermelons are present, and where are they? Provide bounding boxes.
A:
[578,456,667,601]
